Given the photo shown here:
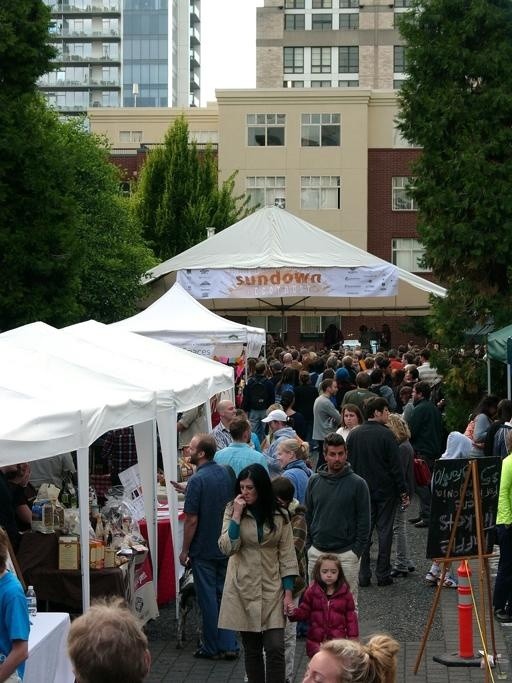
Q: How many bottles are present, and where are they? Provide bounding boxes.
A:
[94,514,105,540]
[59,477,72,510]
[24,585,38,628]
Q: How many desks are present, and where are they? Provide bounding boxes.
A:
[21,601,80,682]
[152,474,192,507]
[124,497,201,609]
[16,527,170,636]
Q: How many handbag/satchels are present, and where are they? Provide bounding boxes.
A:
[412,457,430,485]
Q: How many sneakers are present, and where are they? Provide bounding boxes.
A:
[437,575,458,588]
[425,570,439,584]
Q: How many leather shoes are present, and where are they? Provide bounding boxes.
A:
[494,608,511,623]
[415,520,426,526]
[408,517,419,523]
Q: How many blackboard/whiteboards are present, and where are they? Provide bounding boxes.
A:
[425,455,503,559]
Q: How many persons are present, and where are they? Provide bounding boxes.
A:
[0,526,31,683]
[0,322,511,663]
[301,632,400,682]
[216,462,300,682]
[65,592,152,683]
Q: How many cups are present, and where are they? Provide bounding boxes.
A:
[495,658,509,679]
[41,504,56,534]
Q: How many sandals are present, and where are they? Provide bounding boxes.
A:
[192,647,221,661]
[223,651,238,661]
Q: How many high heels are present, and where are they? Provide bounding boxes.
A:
[391,569,408,577]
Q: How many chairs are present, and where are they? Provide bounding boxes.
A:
[46,3,118,111]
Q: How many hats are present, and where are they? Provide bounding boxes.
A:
[261,409,290,424]
